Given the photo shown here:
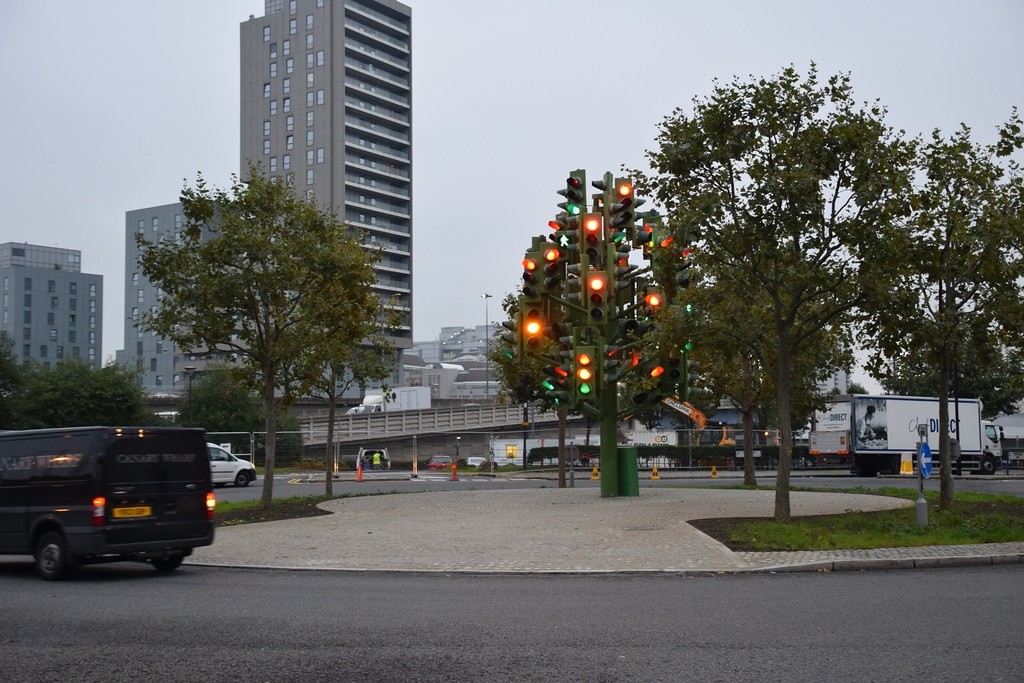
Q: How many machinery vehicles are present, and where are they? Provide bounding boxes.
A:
[660,396,736,470]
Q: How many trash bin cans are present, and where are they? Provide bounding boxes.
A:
[617,446,640,496]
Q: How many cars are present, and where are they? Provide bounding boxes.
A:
[423,454,453,471]
[204,441,257,487]
[464,456,489,469]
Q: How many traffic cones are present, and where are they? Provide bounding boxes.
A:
[449,463,461,482]
[356,465,366,482]
[651,466,660,480]
[710,466,719,479]
[591,467,601,480]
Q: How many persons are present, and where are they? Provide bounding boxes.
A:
[373,450,388,470]
[856,405,876,439]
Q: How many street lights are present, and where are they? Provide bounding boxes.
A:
[481,294,493,400]
[183,366,196,425]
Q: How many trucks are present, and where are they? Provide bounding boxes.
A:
[808,393,1005,478]
[345,386,432,415]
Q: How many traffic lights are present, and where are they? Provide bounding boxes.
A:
[523,303,545,354]
[575,345,596,399]
[522,251,543,304]
[539,241,562,294]
[587,269,608,328]
[500,316,521,364]
[517,167,696,412]
[599,337,621,392]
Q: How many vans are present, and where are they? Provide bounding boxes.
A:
[1,425,220,578]
[356,447,391,471]
